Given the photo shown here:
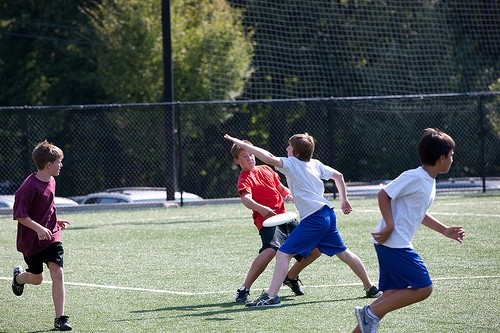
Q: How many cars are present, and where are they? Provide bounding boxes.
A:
[0,194,78,209]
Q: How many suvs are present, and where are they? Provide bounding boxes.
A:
[80,186,203,205]
[438,176,500,189]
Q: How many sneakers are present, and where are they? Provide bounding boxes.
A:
[363,286,382,297]
[236,286,250,304]
[355,305,381,333]
[245,289,282,308]
[283,274,305,296]
[54,315,73,332]
[11,266,25,296]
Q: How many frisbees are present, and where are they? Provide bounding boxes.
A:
[262,212,298,227]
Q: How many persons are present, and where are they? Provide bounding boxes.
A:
[230,140,322,305]
[224,133,383,307]
[11,141,71,330]
[355,128,465,333]
